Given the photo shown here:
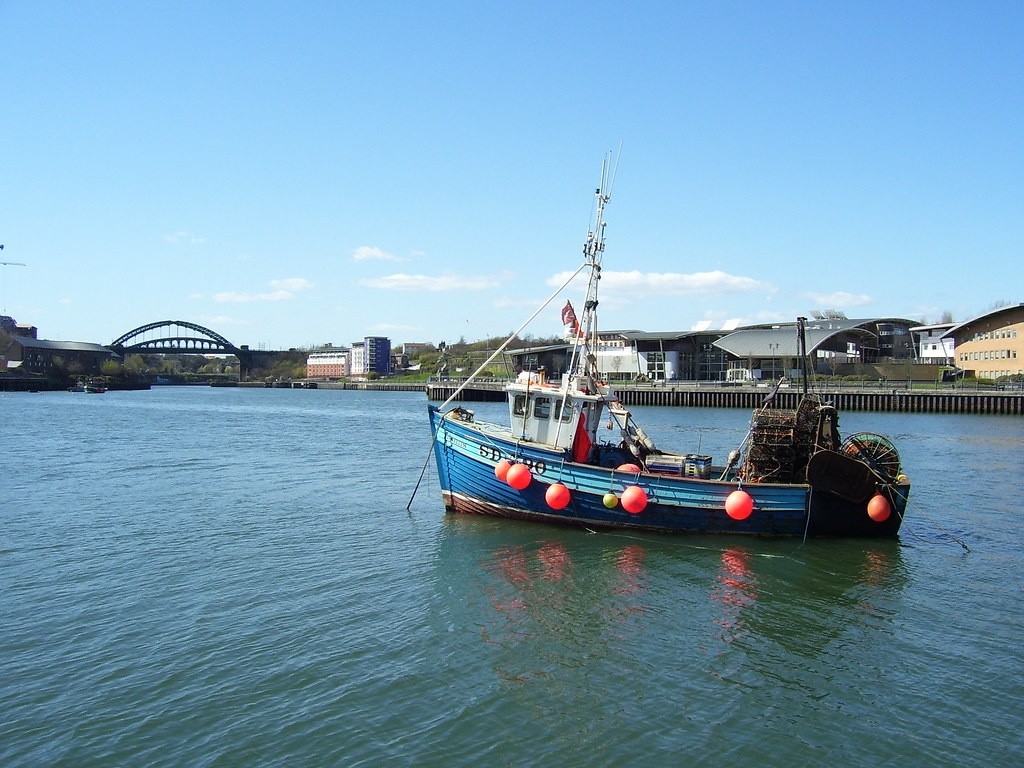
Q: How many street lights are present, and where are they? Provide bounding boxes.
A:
[769,342,780,382]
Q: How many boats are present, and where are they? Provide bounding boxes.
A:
[83,377,106,393]
[427,137,910,539]
[67,379,84,392]
[28,385,39,392]
